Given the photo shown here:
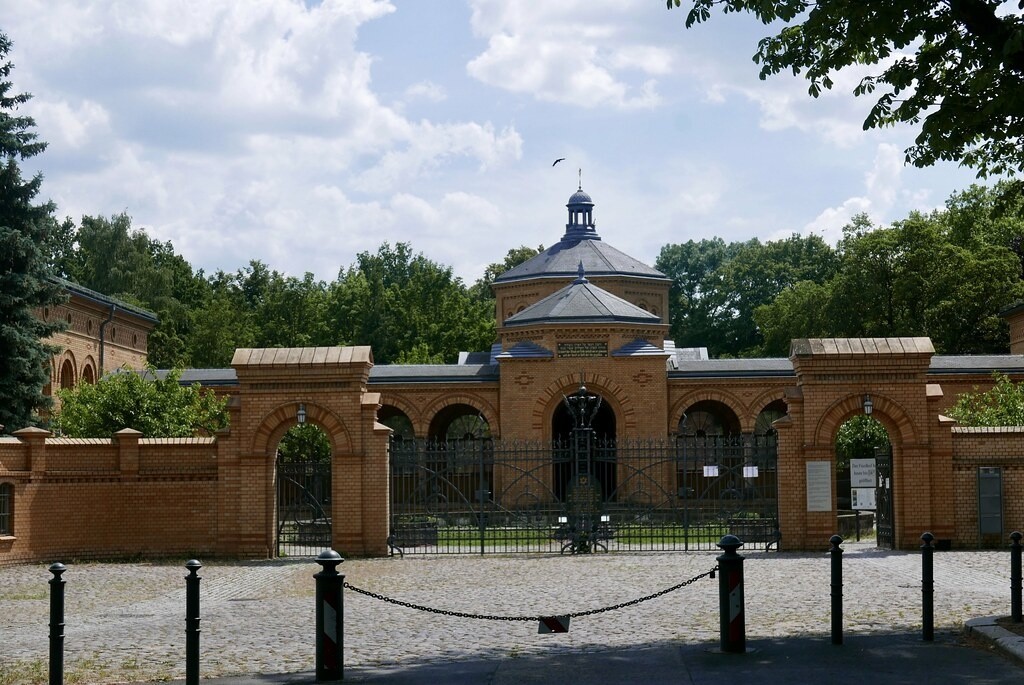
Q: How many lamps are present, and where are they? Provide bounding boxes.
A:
[296,403,306,424]
[864,395,873,416]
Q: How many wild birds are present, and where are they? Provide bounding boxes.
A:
[552,158,565,167]
